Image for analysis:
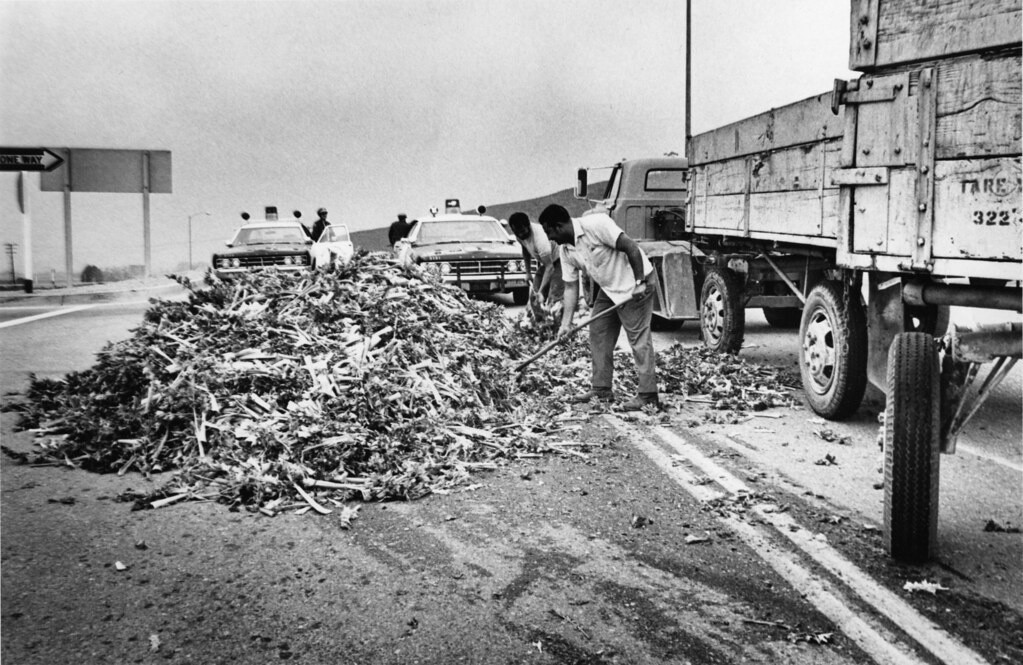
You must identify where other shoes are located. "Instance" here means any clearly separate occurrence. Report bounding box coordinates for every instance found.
[573,387,614,402]
[620,395,658,410]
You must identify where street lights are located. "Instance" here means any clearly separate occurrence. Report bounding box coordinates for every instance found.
[189,211,210,270]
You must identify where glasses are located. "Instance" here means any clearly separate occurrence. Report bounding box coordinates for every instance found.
[321,212,328,214]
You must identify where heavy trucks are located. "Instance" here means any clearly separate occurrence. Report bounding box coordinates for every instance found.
[575,89,951,423]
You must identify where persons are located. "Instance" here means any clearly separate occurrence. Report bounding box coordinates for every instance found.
[388,213,418,243]
[312,208,335,244]
[538,202,660,411]
[507,210,562,326]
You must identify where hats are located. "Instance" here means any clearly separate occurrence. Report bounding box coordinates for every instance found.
[317,208,327,214]
[398,213,407,218]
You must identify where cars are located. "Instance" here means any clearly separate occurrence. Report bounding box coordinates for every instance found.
[210,221,354,279]
[394,214,526,306]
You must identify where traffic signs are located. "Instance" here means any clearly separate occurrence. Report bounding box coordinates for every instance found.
[0,147,65,172]
